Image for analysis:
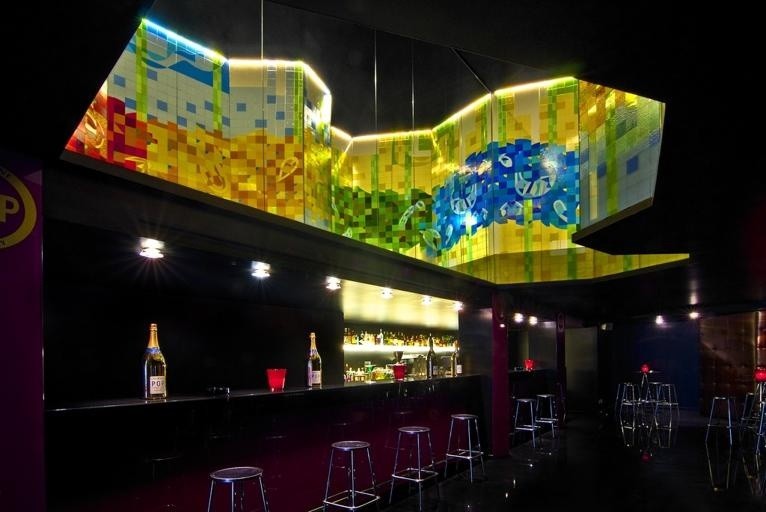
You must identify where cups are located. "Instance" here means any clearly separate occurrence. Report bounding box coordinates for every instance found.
[524,358,535,370]
[266,367,288,393]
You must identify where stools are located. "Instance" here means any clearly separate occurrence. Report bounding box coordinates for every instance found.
[706,391,766,436]
[323,411,486,511]
[615,381,680,416]
[513,393,561,448]
[207,466,269,512]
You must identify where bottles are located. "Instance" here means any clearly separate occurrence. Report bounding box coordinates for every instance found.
[305,331,323,387]
[140,323,168,400]
[342,325,461,383]
